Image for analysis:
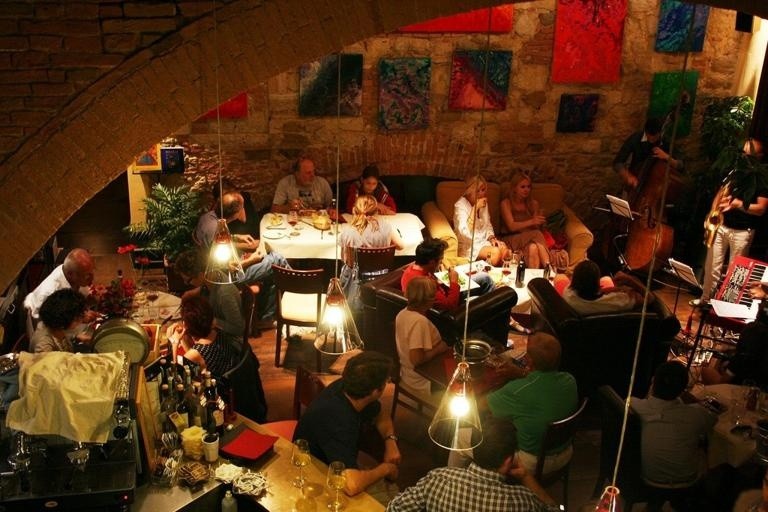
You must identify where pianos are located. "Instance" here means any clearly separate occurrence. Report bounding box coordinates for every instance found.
[708,255,768,327]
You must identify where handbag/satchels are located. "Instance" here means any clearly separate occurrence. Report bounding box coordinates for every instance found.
[554,230,569,251]
[545,208,568,230]
[542,230,555,251]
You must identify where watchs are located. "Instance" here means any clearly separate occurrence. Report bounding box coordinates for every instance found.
[233,234,236,241]
[169,336,179,344]
[383,434,398,441]
[71,332,78,345]
[519,368,528,377]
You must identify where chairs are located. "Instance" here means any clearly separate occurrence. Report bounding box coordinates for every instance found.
[533,397,589,512]
[173,249,263,289]
[596,384,704,511]
[235,283,256,346]
[128,244,165,280]
[257,364,327,443]
[270,263,327,367]
[345,244,396,283]
[389,359,437,429]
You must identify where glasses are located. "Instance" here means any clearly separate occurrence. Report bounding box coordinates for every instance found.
[183,277,194,287]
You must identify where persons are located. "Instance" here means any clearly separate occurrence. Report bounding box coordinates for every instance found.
[401,237,461,310]
[346,163,397,215]
[477,333,577,475]
[166,292,243,395]
[612,117,683,187]
[624,360,719,512]
[270,159,337,217]
[454,174,507,267]
[721,281,768,384]
[689,137,768,306]
[500,172,550,269]
[292,351,402,497]
[338,195,405,313]
[173,252,244,328]
[210,176,259,246]
[29,248,94,330]
[28,288,88,353]
[385,416,560,512]
[394,276,449,397]
[553,260,655,315]
[196,191,293,330]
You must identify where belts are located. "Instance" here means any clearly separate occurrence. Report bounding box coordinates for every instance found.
[519,440,580,457]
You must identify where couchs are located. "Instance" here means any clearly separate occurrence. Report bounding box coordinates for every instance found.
[525,272,679,398]
[358,260,517,352]
[422,181,593,271]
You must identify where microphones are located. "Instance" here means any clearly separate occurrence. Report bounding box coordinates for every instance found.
[722,168,739,183]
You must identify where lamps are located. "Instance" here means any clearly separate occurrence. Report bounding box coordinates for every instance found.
[313,47,361,354]
[592,1,699,510]
[426,3,493,451]
[203,6,245,284]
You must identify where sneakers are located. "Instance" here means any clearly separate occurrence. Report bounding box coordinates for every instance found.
[689,299,704,305]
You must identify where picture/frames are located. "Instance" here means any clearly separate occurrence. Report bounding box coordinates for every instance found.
[132,142,161,171]
[135,367,160,480]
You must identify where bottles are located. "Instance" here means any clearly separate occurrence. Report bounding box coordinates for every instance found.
[221,490,238,512]
[543,262,550,282]
[116,269,123,284]
[330,199,337,222]
[484,252,492,272]
[515,255,526,288]
[159,358,224,437]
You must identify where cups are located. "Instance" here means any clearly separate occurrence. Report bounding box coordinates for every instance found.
[287,210,298,236]
[289,438,311,491]
[145,284,160,310]
[325,461,347,511]
[501,261,511,284]
[202,433,220,465]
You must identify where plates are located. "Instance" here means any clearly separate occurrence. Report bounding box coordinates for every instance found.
[262,228,286,239]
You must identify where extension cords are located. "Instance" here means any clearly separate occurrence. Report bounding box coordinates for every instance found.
[695,352,713,364]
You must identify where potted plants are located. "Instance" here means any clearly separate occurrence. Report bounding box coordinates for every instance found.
[125,179,203,290]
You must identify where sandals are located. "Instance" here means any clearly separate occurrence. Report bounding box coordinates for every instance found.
[526,325,544,335]
[509,318,525,332]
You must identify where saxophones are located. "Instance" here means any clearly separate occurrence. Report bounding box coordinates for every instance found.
[705,182,732,248]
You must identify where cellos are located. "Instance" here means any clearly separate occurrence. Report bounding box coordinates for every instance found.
[625,91,690,270]
